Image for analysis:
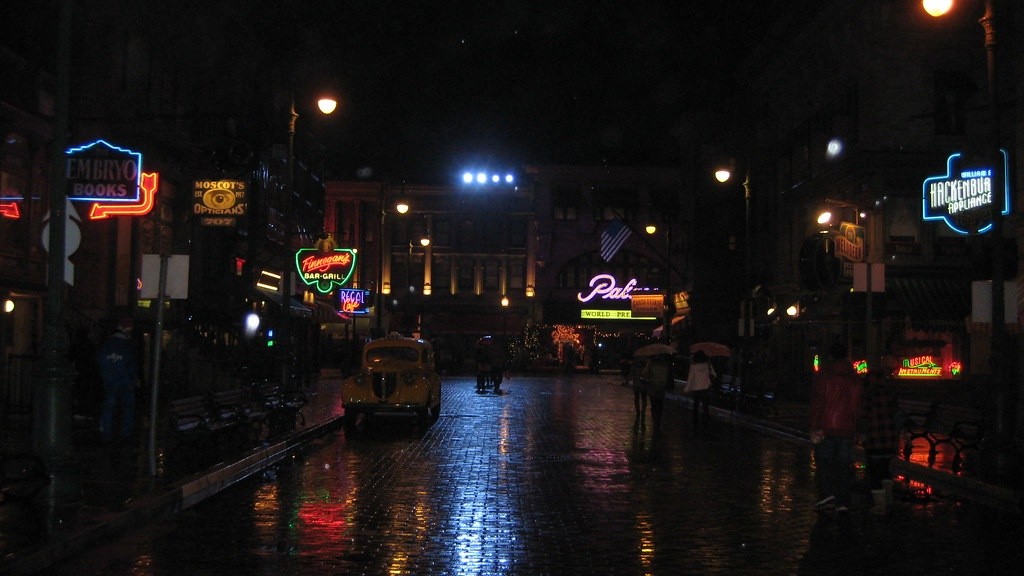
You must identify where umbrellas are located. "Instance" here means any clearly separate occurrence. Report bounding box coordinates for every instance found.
[689,342,732,363]
[633,343,678,361]
[478,336,496,343]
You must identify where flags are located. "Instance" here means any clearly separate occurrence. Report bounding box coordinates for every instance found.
[600,218,632,262]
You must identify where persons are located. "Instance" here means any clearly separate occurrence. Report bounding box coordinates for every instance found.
[96,316,144,454]
[683,350,717,435]
[473,341,506,395]
[627,354,651,423]
[640,351,678,429]
[808,352,860,513]
[855,366,901,516]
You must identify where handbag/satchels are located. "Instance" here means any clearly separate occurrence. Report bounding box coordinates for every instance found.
[666,373,675,393]
[708,361,720,393]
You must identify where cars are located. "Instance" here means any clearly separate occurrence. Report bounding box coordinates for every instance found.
[342,336,442,435]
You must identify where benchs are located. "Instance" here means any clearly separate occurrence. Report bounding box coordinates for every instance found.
[895,398,984,472]
[0,451,53,539]
[715,373,778,416]
[167,379,309,472]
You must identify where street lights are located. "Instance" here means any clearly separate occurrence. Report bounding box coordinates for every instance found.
[373,169,412,334]
[644,216,674,342]
[919,1,1012,480]
[714,150,754,378]
[282,76,339,410]
